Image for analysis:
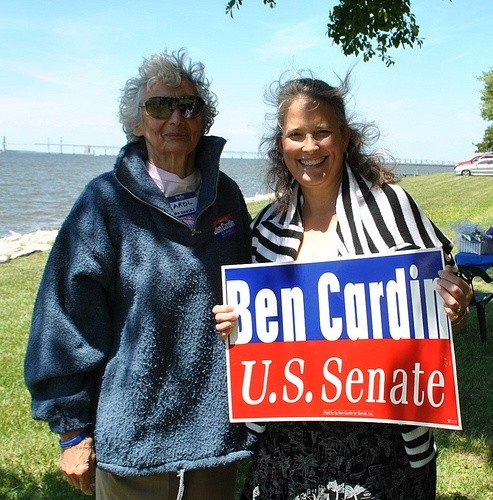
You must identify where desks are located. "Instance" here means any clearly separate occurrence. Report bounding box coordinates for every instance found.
[454,253,493,270]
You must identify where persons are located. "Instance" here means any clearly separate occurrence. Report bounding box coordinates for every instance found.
[211,62,474,500]
[22,47,260,500]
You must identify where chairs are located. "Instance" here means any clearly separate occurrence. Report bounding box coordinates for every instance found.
[455,266,493,344]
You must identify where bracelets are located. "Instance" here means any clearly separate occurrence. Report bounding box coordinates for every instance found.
[59,429,88,449]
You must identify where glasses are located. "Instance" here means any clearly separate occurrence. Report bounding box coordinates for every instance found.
[140,95,206,120]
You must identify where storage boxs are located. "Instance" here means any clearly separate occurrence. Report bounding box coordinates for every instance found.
[459,238,491,255]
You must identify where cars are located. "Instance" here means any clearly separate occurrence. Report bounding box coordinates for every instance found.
[455,155,493,165]
[455,159,493,177]
